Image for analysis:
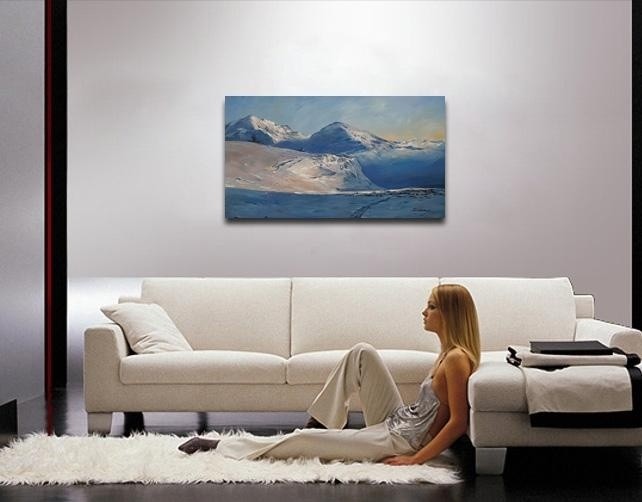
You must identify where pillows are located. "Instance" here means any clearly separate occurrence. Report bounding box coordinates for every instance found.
[99,298,194,358]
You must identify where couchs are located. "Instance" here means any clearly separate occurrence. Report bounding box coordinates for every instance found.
[80,273,641,474]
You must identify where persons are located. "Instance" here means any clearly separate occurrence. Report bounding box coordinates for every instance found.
[176,282,482,466]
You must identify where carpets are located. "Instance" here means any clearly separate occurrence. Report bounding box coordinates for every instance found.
[1,429,465,488]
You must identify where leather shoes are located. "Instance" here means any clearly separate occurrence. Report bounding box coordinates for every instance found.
[178,437,221,454]
